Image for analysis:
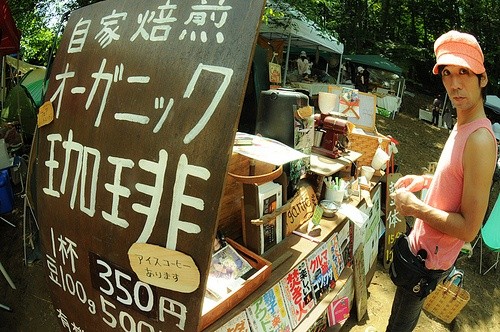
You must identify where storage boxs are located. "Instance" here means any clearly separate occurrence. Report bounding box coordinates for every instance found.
[0,168,14,213]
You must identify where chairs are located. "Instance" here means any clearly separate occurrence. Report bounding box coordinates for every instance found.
[472,190,500,275]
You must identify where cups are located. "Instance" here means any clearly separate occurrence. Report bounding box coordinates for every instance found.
[317,92,337,114]
[371,146,390,170]
[320,199,339,218]
[325,187,345,204]
[361,165,376,181]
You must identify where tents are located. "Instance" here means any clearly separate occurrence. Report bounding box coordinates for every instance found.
[484,95,500,114]
[258,0,344,86]
[341,55,404,120]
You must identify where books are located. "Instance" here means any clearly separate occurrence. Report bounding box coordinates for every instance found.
[213,233,350,332]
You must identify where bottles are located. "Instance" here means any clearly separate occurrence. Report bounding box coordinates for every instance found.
[258,87,310,149]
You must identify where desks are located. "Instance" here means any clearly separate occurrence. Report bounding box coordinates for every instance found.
[419,108,443,128]
[292,82,330,104]
[376,93,402,120]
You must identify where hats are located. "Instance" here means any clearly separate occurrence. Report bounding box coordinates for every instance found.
[300,51,306,57]
[433,30,486,75]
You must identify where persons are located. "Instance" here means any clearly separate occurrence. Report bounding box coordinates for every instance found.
[430,95,442,127]
[297,51,310,78]
[355,66,365,92]
[386,29,498,332]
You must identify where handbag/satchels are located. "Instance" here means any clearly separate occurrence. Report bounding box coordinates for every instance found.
[385,227,428,289]
[421,273,471,324]
